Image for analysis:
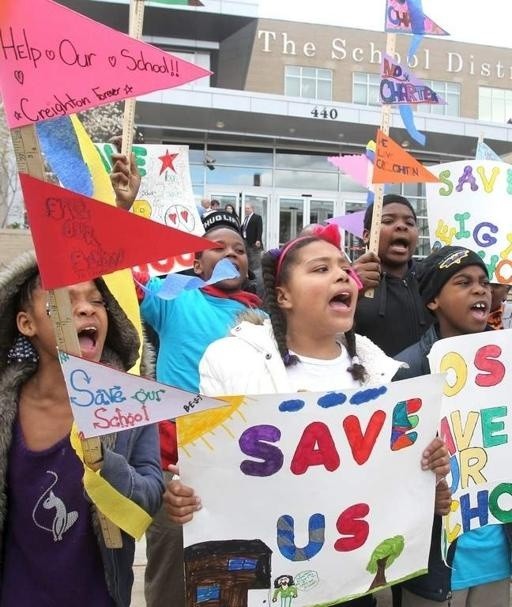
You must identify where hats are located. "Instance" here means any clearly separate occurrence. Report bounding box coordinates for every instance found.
[417,245,489,316]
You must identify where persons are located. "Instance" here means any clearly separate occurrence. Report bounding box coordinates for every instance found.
[391,246,512,607]
[109,153,270,607]
[300,194,512,358]
[0,249,166,607]
[163,236,450,607]
